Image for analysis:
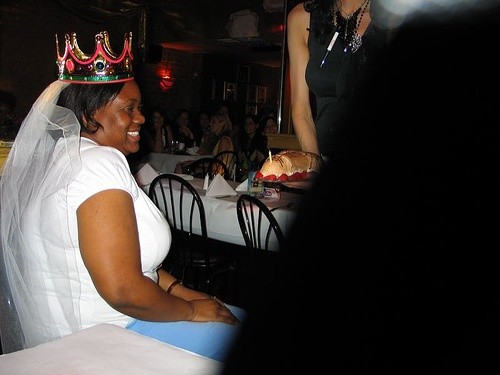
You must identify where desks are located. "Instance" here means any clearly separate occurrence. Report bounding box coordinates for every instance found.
[136,168,299,254]
[148,150,213,173]
[0,322,224,375]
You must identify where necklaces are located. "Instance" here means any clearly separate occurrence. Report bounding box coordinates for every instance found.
[332,0,370,50]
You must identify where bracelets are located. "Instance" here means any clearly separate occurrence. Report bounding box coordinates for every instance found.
[167,279,180,294]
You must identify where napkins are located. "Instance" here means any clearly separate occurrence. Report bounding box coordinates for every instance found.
[204,168,240,200]
[134,162,160,187]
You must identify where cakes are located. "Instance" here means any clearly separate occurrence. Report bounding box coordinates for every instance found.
[255,150,323,181]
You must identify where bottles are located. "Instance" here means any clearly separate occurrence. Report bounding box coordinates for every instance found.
[248,157,264,199]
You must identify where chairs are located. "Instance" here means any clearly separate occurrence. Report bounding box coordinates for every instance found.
[183,157,230,180]
[234,194,285,259]
[208,150,238,179]
[148,173,223,299]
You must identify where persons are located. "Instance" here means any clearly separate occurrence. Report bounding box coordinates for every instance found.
[0,90,26,139]
[0,78,246,363]
[145,0,500,375]
